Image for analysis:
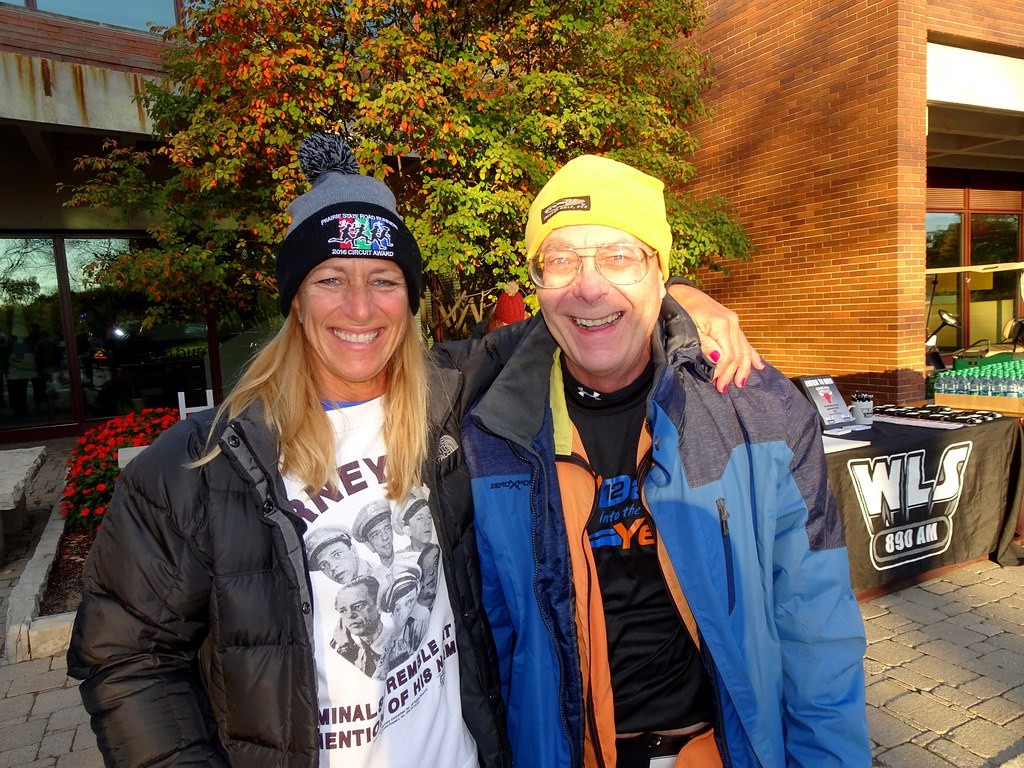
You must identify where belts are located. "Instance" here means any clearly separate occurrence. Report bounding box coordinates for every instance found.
[650,725,712,758]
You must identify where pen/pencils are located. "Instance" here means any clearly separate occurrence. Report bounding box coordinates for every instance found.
[851,390,873,402]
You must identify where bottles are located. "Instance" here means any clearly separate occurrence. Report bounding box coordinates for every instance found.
[934,359,1024,396]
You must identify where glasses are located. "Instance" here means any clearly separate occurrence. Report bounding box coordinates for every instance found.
[524,244,658,290]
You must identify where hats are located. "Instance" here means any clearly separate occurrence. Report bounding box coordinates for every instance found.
[526,155,672,284]
[275,133,423,316]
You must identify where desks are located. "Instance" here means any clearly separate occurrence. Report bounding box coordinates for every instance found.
[819,404,1024,604]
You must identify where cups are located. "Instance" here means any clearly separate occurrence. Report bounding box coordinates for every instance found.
[847,400,874,425]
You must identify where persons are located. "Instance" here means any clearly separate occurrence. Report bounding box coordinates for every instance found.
[66,172,765,768]
[460,154,873,768]
[471,293,532,339]
[0,320,103,421]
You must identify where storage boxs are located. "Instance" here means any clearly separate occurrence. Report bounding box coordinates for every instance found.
[934,393,1024,419]
